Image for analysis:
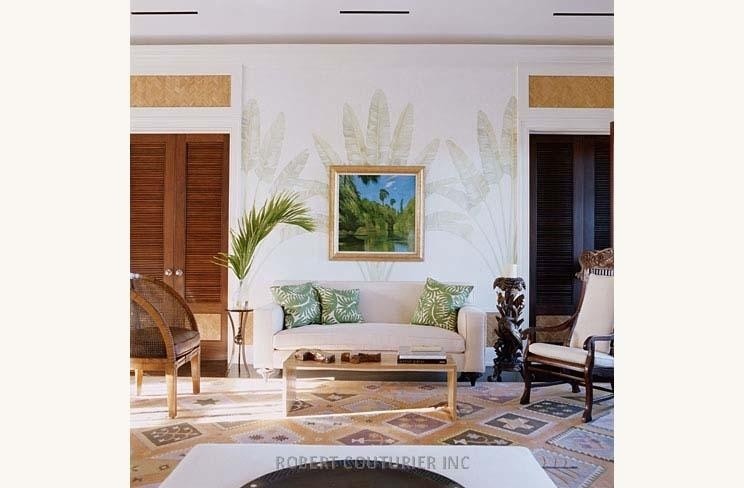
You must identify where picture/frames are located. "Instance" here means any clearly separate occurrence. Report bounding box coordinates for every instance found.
[329,165,425,262]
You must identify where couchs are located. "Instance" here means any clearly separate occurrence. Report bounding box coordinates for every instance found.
[251,280,487,386]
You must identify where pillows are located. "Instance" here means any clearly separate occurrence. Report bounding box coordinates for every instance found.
[310,282,365,324]
[270,283,322,329]
[409,277,474,333]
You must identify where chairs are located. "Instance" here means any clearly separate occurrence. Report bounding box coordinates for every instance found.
[516,247,614,424]
[130,272,202,420]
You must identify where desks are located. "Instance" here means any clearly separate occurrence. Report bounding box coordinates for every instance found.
[158,443,558,488]
[224,307,254,378]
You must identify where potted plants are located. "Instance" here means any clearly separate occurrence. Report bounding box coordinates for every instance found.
[206,187,320,309]
